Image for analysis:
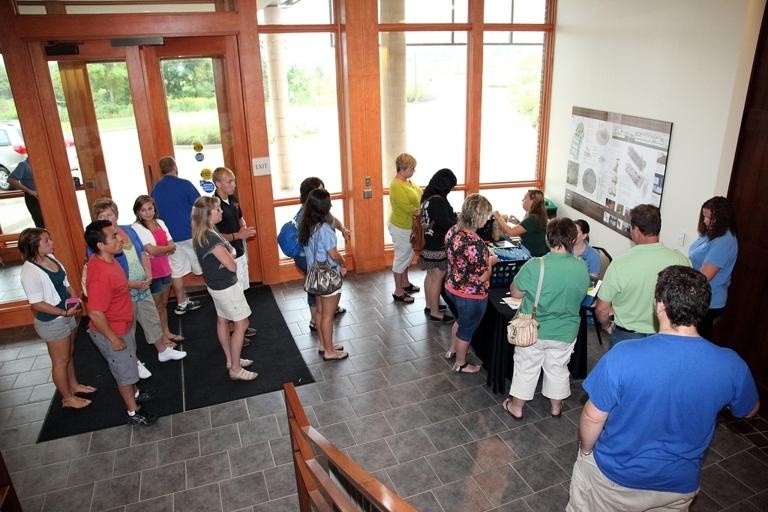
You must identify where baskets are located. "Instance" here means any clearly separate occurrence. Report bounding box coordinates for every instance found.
[489,245,533,287]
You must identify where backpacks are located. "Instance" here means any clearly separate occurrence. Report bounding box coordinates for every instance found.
[410,195,440,251]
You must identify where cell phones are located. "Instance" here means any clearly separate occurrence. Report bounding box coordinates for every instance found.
[67,302,81,310]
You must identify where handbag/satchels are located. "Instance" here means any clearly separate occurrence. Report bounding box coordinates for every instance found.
[505,258,545,348]
[303,236,343,296]
[277,208,305,258]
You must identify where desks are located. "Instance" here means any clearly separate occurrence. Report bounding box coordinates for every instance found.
[437,219,590,393]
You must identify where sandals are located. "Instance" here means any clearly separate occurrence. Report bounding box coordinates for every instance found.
[309,321,317,330]
[451,362,481,374]
[335,305,346,318]
[445,346,472,359]
[72,385,97,394]
[61,396,93,409]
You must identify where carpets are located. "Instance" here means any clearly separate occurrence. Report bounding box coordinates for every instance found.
[181,282,324,414]
[31,302,185,445]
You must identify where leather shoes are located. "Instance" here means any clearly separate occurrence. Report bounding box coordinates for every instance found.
[324,351,349,361]
[319,344,343,354]
[425,305,447,313]
[430,314,454,324]
[225,358,254,370]
[229,368,258,381]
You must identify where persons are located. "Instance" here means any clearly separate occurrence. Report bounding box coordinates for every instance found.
[595,205,693,350]
[131,195,185,350]
[85,220,158,425]
[388,153,423,303]
[492,190,550,257]
[86,198,187,379]
[420,169,459,324]
[212,167,257,347]
[298,188,348,361]
[502,217,589,420]
[151,157,204,316]
[688,197,739,345]
[7,157,46,227]
[444,194,497,374]
[18,228,97,408]
[191,197,258,382]
[573,220,601,325]
[294,177,352,332]
[566,265,760,512]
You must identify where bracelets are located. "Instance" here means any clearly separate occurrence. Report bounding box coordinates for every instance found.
[581,448,592,456]
[231,233,234,241]
[66,310,67,317]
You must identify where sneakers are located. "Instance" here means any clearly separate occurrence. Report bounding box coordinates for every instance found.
[174,298,202,314]
[135,387,158,404]
[136,359,153,380]
[123,407,157,426]
[245,325,257,336]
[157,348,187,362]
[243,337,252,350]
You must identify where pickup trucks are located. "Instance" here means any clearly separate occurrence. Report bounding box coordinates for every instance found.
[0,120,84,192]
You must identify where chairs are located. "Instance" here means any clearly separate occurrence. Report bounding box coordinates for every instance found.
[581,246,615,345]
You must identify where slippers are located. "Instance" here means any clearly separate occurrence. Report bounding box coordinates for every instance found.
[404,282,420,292]
[502,398,524,422]
[392,292,415,303]
[169,333,185,344]
[167,340,178,349]
[551,401,564,418]
